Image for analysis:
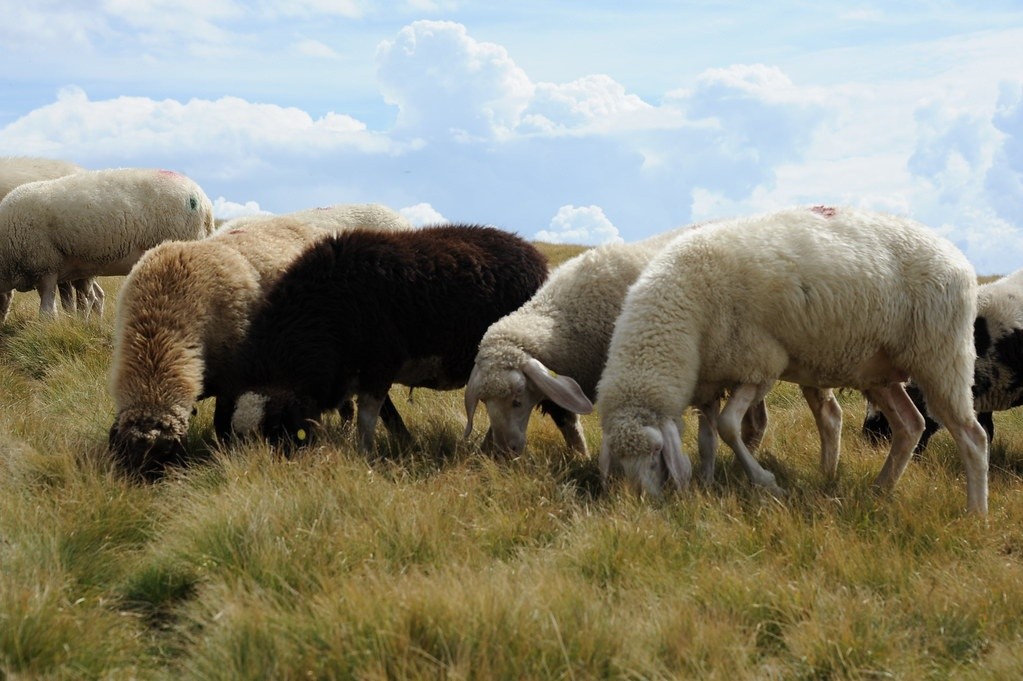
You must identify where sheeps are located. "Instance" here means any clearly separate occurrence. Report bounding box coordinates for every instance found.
[0,169,220,325]
[595,212,989,528]
[228,227,588,466]
[466,219,843,488]
[0,157,87,326]
[110,202,414,487]
[861,267,1023,466]
